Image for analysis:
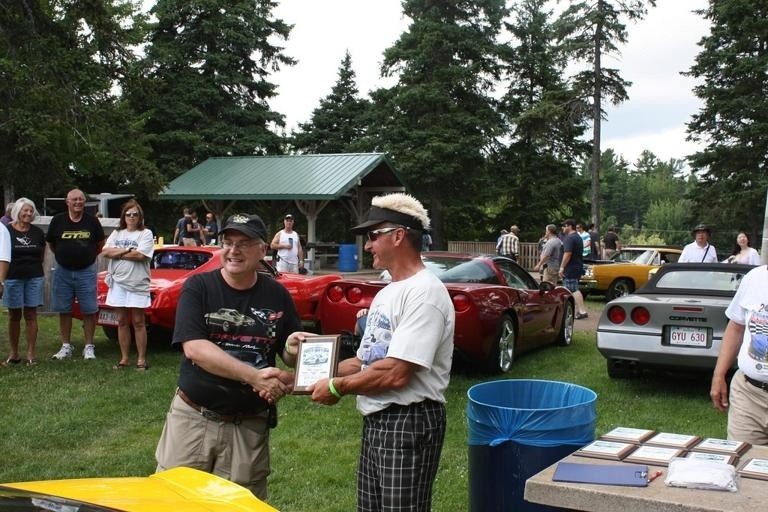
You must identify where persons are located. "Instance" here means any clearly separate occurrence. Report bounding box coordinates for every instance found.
[0,219,12,299]
[719,230,761,266]
[250,189,458,511]
[153,211,323,504]
[269,212,304,275]
[0,196,45,366]
[45,186,106,363]
[709,258,768,447]
[533,220,622,320]
[498,229,508,256]
[172,207,220,249]
[0,202,16,225]
[99,198,154,369]
[495,224,521,266]
[676,224,720,263]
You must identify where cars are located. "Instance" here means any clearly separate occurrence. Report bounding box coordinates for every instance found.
[0,465,281,511]
[556,242,684,301]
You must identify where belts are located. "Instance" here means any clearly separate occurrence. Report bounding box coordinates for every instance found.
[177,388,259,423]
[744,375,768,392]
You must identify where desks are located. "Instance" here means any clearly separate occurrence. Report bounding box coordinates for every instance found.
[522,426,768,512]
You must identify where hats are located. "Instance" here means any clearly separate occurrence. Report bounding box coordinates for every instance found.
[284,214,294,220]
[350,205,423,235]
[217,212,267,242]
[691,224,712,239]
[510,225,520,232]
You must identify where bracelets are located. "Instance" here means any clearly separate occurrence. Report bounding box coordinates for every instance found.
[298,258,304,264]
[283,337,290,353]
[126,245,134,253]
[0,280,5,286]
[328,377,343,399]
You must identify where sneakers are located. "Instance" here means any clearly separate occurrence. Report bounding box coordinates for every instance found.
[81,345,96,359]
[575,311,588,319]
[51,346,72,360]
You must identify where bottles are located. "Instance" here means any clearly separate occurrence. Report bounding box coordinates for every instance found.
[153,236,157,244]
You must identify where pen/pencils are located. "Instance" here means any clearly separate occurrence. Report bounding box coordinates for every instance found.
[648,471,662,482]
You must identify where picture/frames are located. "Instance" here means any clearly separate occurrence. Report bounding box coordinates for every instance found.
[572,427,768,481]
[291,334,342,396]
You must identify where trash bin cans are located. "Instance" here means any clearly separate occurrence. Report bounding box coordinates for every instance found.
[466,379,598,512]
[338,244,359,272]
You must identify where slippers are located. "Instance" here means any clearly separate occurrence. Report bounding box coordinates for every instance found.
[1,357,37,368]
[114,360,147,373]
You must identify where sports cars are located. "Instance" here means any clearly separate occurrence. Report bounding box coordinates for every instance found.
[592,262,762,381]
[66,240,346,350]
[307,249,581,377]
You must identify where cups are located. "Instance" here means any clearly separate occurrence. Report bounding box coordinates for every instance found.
[158,237,164,247]
[288,238,293,245]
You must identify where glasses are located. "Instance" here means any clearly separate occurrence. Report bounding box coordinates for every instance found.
[367,227,409,241]
[220,240,259,250]
[125,211,138,218]
